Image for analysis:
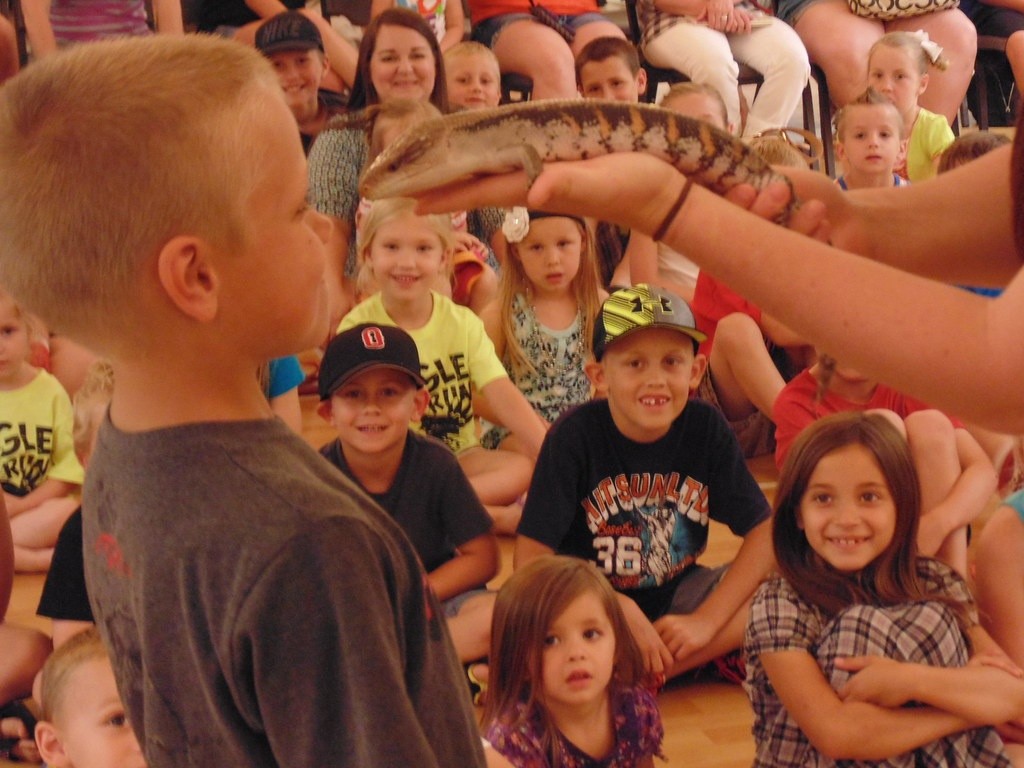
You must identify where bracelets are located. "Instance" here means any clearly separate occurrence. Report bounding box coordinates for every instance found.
[651,176,694,242]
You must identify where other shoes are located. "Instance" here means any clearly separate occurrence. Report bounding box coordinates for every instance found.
[465,664,482,704]
[675,649,747,686]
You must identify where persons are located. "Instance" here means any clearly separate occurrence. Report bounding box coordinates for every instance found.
[0,0,1024,768]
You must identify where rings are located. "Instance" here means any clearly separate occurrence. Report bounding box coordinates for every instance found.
[721,16,727,20]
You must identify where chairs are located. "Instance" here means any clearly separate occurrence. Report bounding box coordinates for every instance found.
[498,0,1024,185]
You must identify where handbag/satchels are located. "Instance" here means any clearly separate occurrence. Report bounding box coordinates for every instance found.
[685,11,772,27]
[848,0,959,21]
[532,3,576,41]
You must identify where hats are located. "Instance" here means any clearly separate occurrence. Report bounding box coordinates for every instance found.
[593,282,708,363]
[319,323,428,400]
[254,12,325,55]
